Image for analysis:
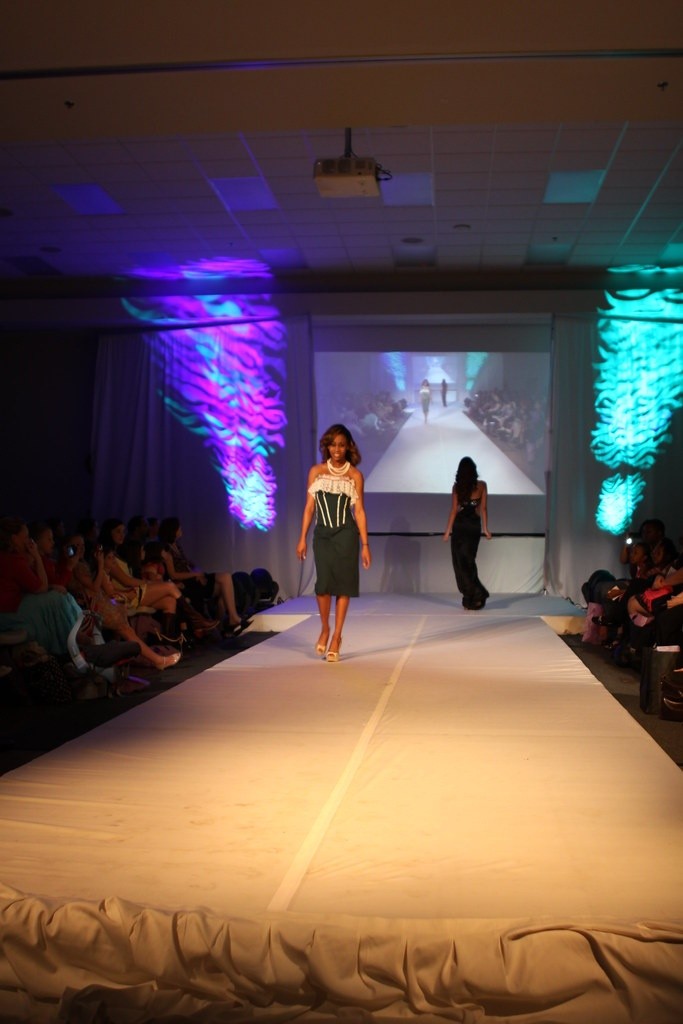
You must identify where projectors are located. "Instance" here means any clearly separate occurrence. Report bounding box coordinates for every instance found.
[313,157,381,198]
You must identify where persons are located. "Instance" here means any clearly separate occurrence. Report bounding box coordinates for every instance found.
[574,520,683,673]
[445,457,492,611]
[297,423,372,661]
[332,389,407,441]
[463,387,546,464]
[419,379,433,417]
[440,380,448,407]
[0,517,288,723]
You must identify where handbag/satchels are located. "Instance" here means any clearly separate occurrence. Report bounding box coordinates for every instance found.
[581,602,609,648]
[20,657,73,705]
[639,647,683,714]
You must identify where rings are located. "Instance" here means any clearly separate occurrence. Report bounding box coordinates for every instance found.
[608,591,611,594]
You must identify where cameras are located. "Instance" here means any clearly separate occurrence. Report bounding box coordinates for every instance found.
[627,532,641,544]
[66,545,77,557]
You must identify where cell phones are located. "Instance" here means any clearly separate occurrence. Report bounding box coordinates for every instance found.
[98,545,105,551]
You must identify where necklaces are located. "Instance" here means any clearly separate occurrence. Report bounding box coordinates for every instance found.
[327,458,350,476]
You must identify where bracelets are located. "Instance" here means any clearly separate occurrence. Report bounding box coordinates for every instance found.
[362,544,368,545]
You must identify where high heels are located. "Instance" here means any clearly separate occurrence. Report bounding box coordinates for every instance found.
[326,637,341,661]
[316,643,329,655]
[155,653,180,672]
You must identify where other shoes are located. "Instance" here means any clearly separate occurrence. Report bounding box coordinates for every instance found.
[187,614,220,634]
[230,619,254,636]
[608,642,624,651]
[1,666,11,677]
[592,615,614,627]
